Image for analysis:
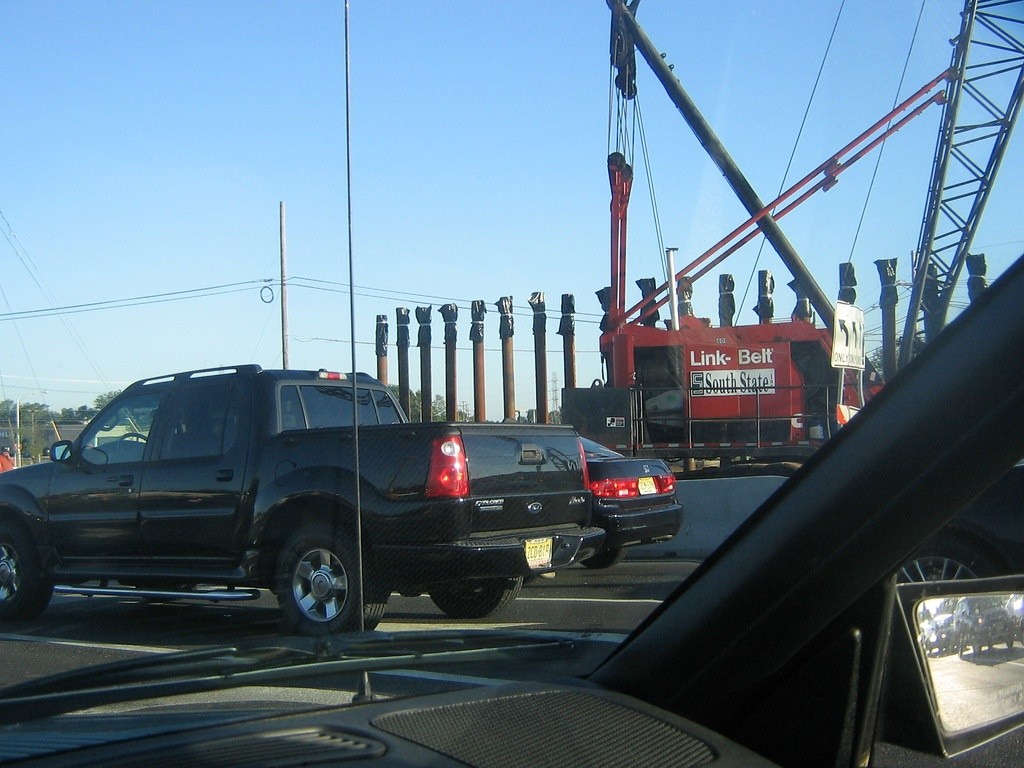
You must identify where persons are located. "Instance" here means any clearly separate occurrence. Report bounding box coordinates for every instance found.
[0,447,14,473]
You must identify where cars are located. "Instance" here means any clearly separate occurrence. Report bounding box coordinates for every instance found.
[575,435,683,570]
[917,593,1024,655]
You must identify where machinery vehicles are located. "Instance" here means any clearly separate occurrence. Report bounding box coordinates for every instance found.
[604,0,884,461]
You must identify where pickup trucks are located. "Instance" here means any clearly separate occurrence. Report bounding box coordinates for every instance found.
[1,364,607,637]
